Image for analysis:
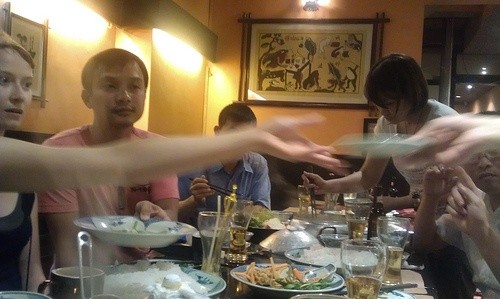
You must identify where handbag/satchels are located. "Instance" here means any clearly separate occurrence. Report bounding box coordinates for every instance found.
[427,246,475,299]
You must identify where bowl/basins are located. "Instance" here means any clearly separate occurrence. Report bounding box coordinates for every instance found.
[72,216,197,248]
[232,212,310,244]
[318,233,349,248]
[269,211,295,222]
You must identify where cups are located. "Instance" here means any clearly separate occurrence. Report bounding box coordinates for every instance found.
[197,199,254,277]
[340,239,387,299]
[298,181,412,291]
[0,290,53,299]
[37,266,122,299]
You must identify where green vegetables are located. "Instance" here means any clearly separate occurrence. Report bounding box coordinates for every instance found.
[233,212,263,228]
[277,268,335,290]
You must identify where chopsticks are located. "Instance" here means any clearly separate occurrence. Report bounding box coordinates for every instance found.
[189,177,244,200]
[310,177,317,217]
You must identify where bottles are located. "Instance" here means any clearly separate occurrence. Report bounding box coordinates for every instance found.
[367,186,386,240]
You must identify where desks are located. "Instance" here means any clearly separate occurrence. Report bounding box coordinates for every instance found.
[220,208,435,299]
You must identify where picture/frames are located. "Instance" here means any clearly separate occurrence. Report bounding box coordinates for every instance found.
[363,118,378,137]
[9,12,49,108]
[236,10,389,108]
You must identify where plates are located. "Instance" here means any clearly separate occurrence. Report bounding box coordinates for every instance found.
[284,246,382,276]
[331,138,425,157]
[230,264,417,299]
[179,266,227,296]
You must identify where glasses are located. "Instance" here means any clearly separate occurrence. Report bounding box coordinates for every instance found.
[466,151,500,165]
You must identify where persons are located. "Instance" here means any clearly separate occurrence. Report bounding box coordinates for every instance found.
[412,111,500,299]
[0,33,49,299]
[406,114,500,168]
[35,48,180,269]
[446,166,500,282]
[301,54,462,216]
[0,117,349,193]
[176,103,272,239]
